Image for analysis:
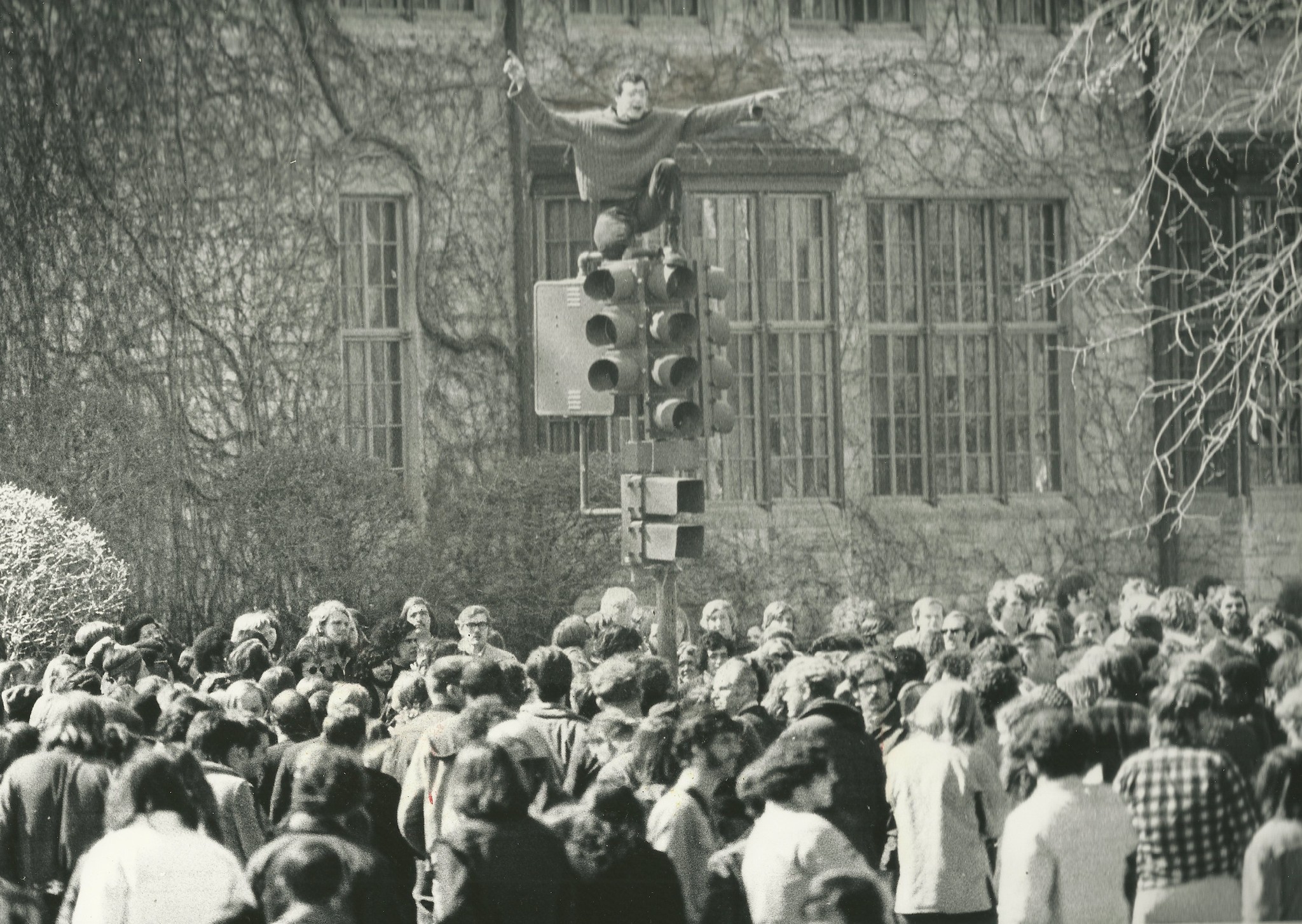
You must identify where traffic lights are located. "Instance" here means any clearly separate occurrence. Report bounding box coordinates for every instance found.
[583,269,639,392]
[646,262,700,435]
[642,476,706,561]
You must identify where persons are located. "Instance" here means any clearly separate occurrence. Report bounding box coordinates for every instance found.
[890,676,1000,922]
[1239,743,1302,920]
[743,572,1302,870]
[503,47,790,266]
[643,709,740,923]
[54,745,261,924]
[424,744,586,922]
[998,712,1138,920]
[573,778,687,924]
[1,690,111,922]
[1114,679,1259,922]
[179,712,263,873]
[2,582,736,856]
[238,743,414,924]
[268,835,353,924]
[738,734,898,924]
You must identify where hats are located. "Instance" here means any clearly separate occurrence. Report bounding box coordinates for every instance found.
[588,654,640,702]
[737,735,831,803]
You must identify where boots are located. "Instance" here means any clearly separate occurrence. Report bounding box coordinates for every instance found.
[659,213,689,268]
